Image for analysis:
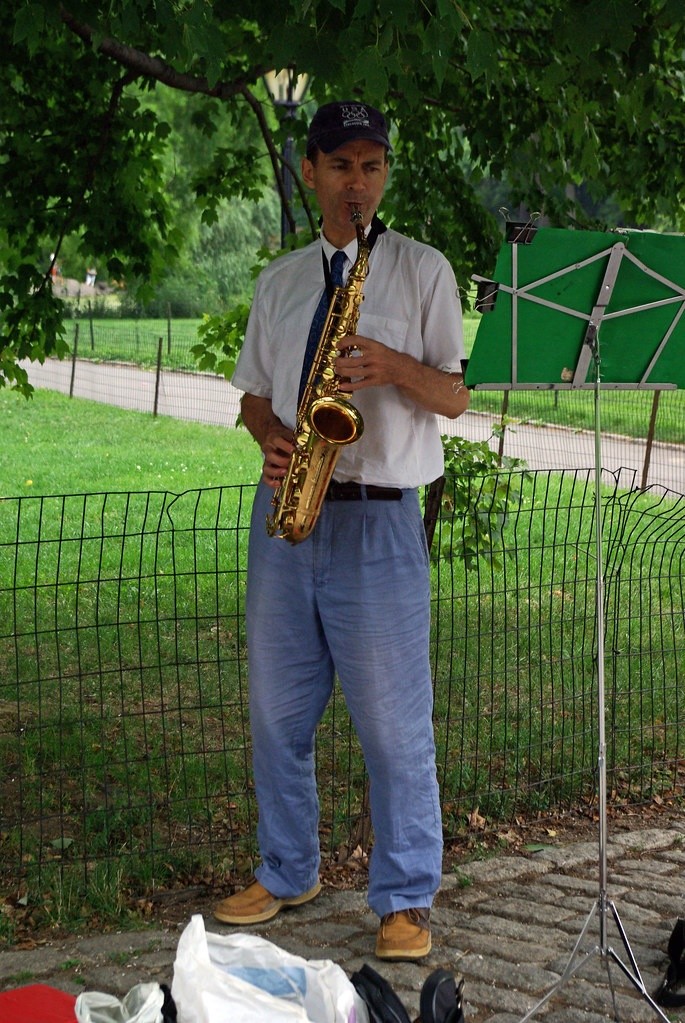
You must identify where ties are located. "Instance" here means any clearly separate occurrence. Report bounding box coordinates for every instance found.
[298,250,348,420]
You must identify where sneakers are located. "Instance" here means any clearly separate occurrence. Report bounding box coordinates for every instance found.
[214,870,321,925]
[374,907,432,960]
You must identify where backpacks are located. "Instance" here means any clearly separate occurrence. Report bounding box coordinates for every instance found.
[351,964,411,1023]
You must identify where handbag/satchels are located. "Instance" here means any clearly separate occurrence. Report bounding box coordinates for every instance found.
[419,967,466,1023]
[74,982,164,1023]
[656,917,685,1007]
[170,915,370,1023]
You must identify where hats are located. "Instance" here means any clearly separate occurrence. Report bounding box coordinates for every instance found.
[305,101,393,159]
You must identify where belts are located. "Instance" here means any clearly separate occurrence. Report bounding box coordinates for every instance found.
[325,480,403,500]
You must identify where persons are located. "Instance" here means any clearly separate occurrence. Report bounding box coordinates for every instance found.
[216,99,471,963]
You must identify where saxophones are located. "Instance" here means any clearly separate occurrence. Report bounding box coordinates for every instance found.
[266,201,387,544]
